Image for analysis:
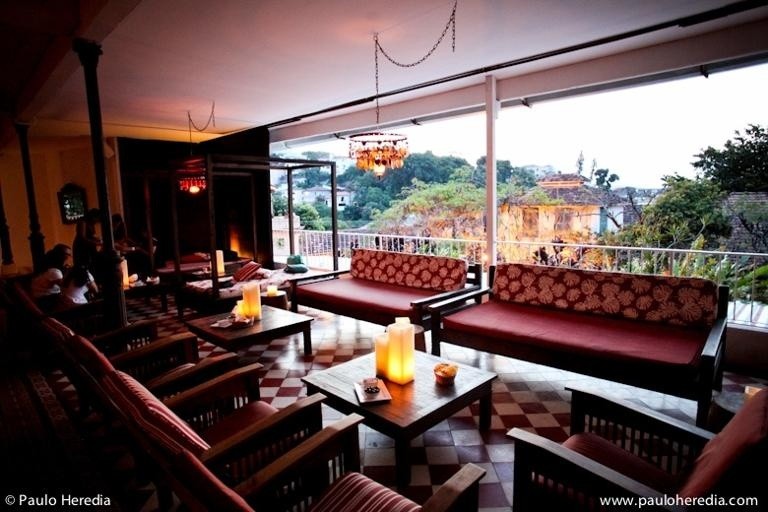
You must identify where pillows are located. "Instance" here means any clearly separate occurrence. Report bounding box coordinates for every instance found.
[284,252,309,274]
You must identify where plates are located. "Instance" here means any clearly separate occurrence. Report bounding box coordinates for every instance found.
[211,321,232,328]
[353,379,393,404]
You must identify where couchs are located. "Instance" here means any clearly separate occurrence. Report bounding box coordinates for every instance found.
[285,245,733,427]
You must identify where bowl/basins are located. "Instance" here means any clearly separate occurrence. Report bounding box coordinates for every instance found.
[434,363,455,386]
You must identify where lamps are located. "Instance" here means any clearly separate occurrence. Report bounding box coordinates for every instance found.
[174,102,217,198]
[346,2,460,180]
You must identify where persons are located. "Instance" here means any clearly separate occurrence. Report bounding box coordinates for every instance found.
[73,208,160,270]
[30,243,99,312]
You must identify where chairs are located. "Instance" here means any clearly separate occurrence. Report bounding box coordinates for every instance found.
[502,374,768,511]
[13,278,497,510]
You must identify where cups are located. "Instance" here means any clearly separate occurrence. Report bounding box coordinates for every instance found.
[363,377,377,388]
